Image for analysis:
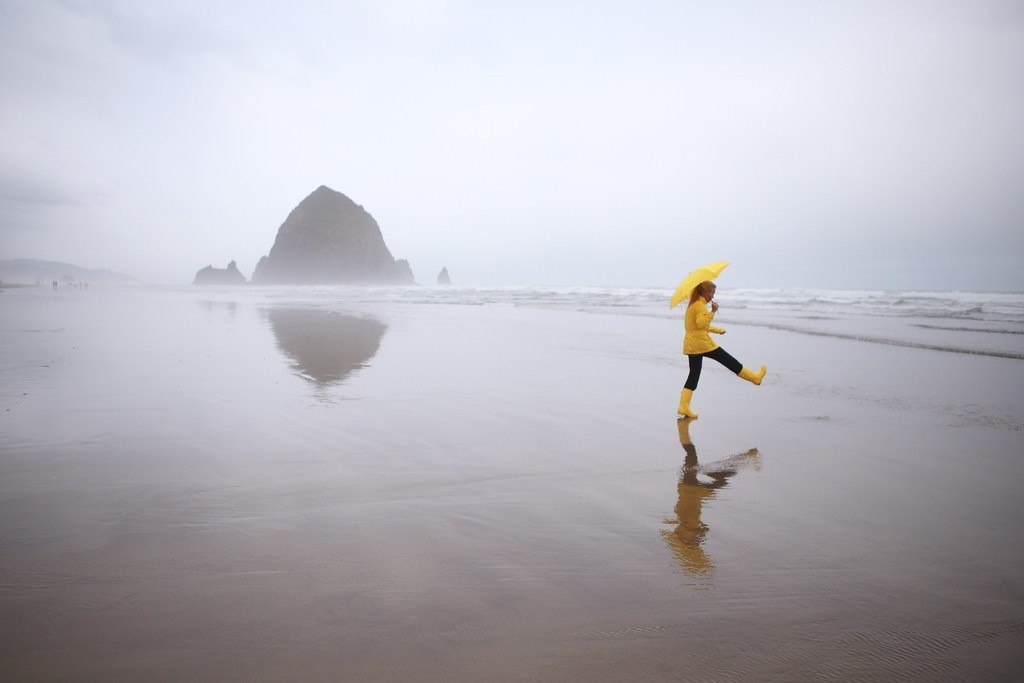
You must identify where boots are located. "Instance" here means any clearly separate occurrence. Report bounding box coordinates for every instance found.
[739,365,767,386]
[677,387,698,418]
[677,416,692,446]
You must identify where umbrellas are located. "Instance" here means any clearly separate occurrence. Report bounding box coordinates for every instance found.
[670,260,730,308]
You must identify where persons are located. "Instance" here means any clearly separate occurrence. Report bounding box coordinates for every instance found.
[677,281,766,418]
